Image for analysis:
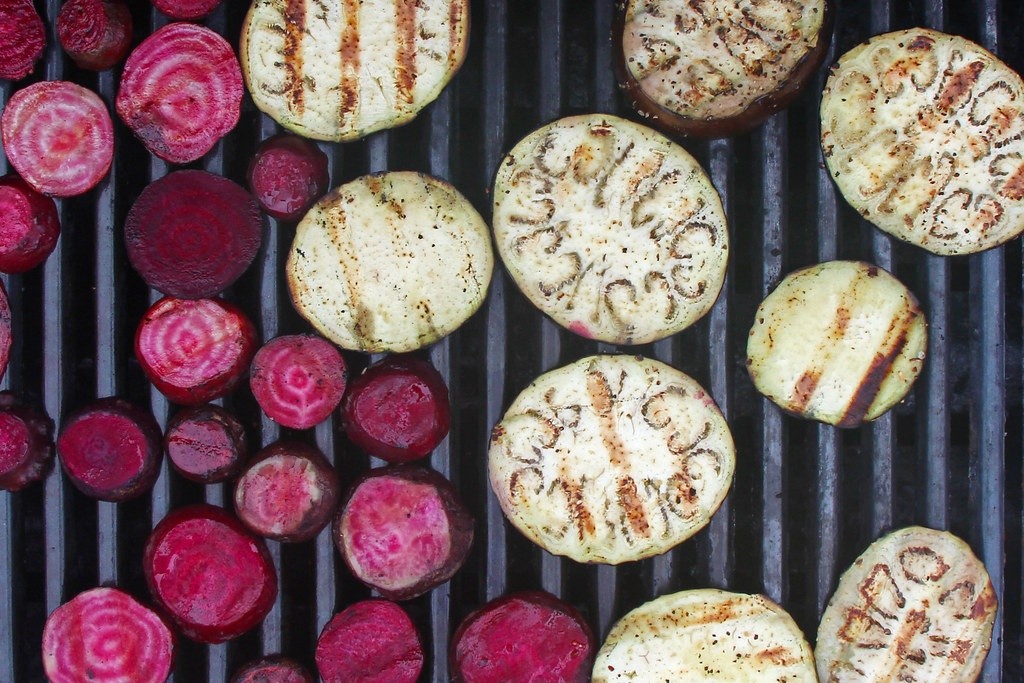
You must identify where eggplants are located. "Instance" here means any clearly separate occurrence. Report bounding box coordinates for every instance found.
[239,0,1024,683]
